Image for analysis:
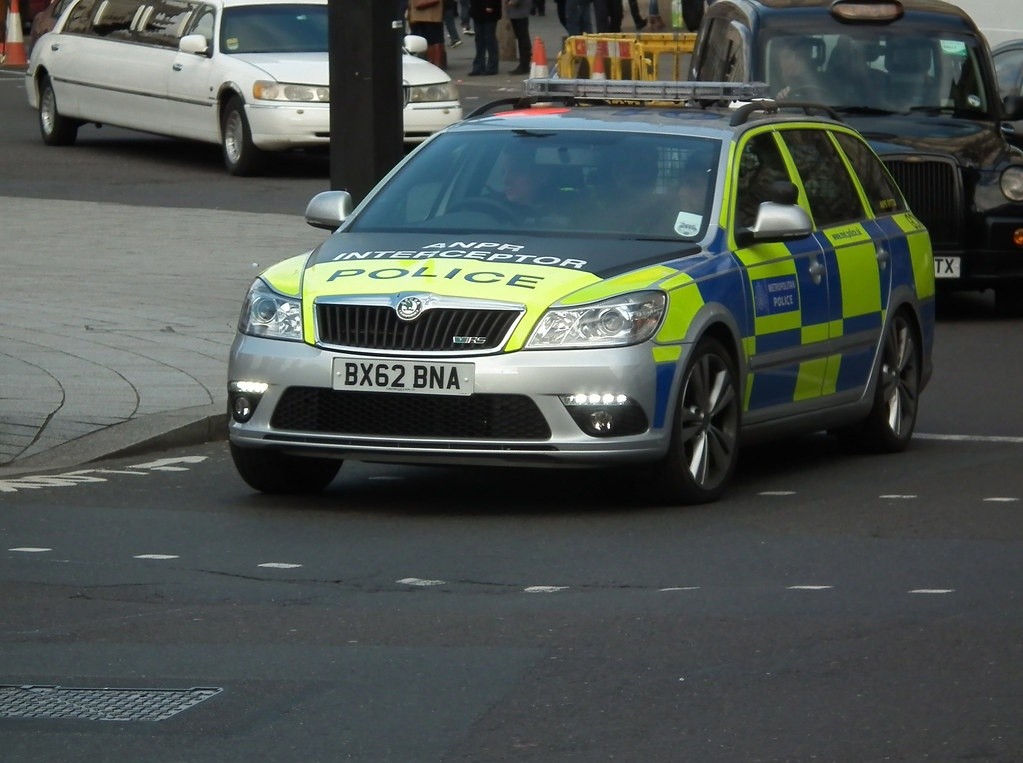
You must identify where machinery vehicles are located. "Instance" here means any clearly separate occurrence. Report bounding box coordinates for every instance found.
[495,32,697,196]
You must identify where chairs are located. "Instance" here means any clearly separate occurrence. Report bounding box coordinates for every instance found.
[883,41,940,109]
[548,166,584,205]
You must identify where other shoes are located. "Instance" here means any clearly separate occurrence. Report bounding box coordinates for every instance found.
[635,19,647,30]
[462,27,475,34]
[481,70,497,75]
[451,38,462,49]
[511,67,530,75]
[468,69,481,77]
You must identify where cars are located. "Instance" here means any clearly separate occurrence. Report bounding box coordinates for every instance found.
[682,1,1022,315]
[25,1,464,177]
[227,79,935,505]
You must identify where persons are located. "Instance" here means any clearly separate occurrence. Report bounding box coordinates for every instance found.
[464,143,572,227]
[554,0,624,35]
[773,39,828,100]
[680,0,706,31]
[638,150,717,233]
[467,0,502,75]
[409,0,443,67]
[504,0,532,74]
[627,0,667,30]
[460,0,473,30]
[443,0,461,48]
[531,0,545,16]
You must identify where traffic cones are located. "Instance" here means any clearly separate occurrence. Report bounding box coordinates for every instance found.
[520,37,552,106]
[588,54,607,77]
[0,0,28,67]
